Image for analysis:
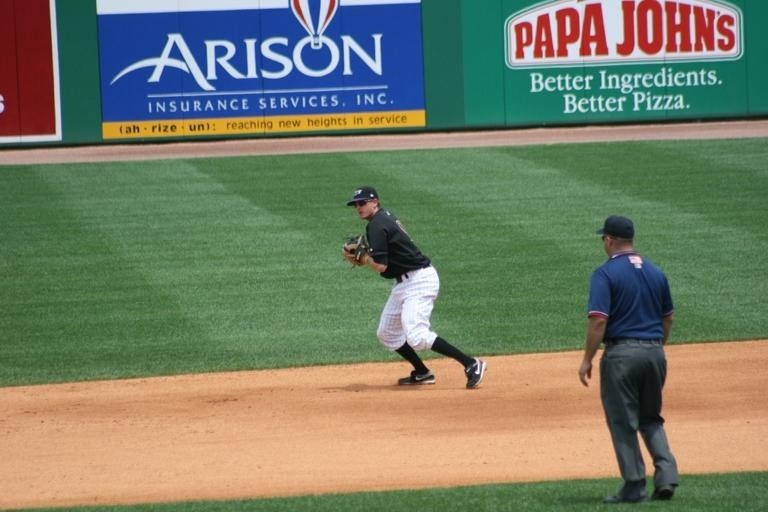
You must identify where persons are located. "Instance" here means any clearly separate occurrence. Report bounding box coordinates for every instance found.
[578,214,679,501]
[339,186,486,388]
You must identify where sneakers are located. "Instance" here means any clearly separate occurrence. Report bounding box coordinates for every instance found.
[465,358,487,388]
[399,369,435,385]
[652,484,674,499]
[604,482,647,502]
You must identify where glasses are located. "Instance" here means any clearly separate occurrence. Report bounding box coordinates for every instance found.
[354,200,372,207]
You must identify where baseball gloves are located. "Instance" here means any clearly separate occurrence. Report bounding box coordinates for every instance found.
[342,234,367,265]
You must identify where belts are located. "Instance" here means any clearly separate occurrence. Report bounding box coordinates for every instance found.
[606,339,664,344]
[396,273,408,283]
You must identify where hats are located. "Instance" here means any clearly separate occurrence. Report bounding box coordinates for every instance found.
[597,216,634,239]
[347,186,378,206]
